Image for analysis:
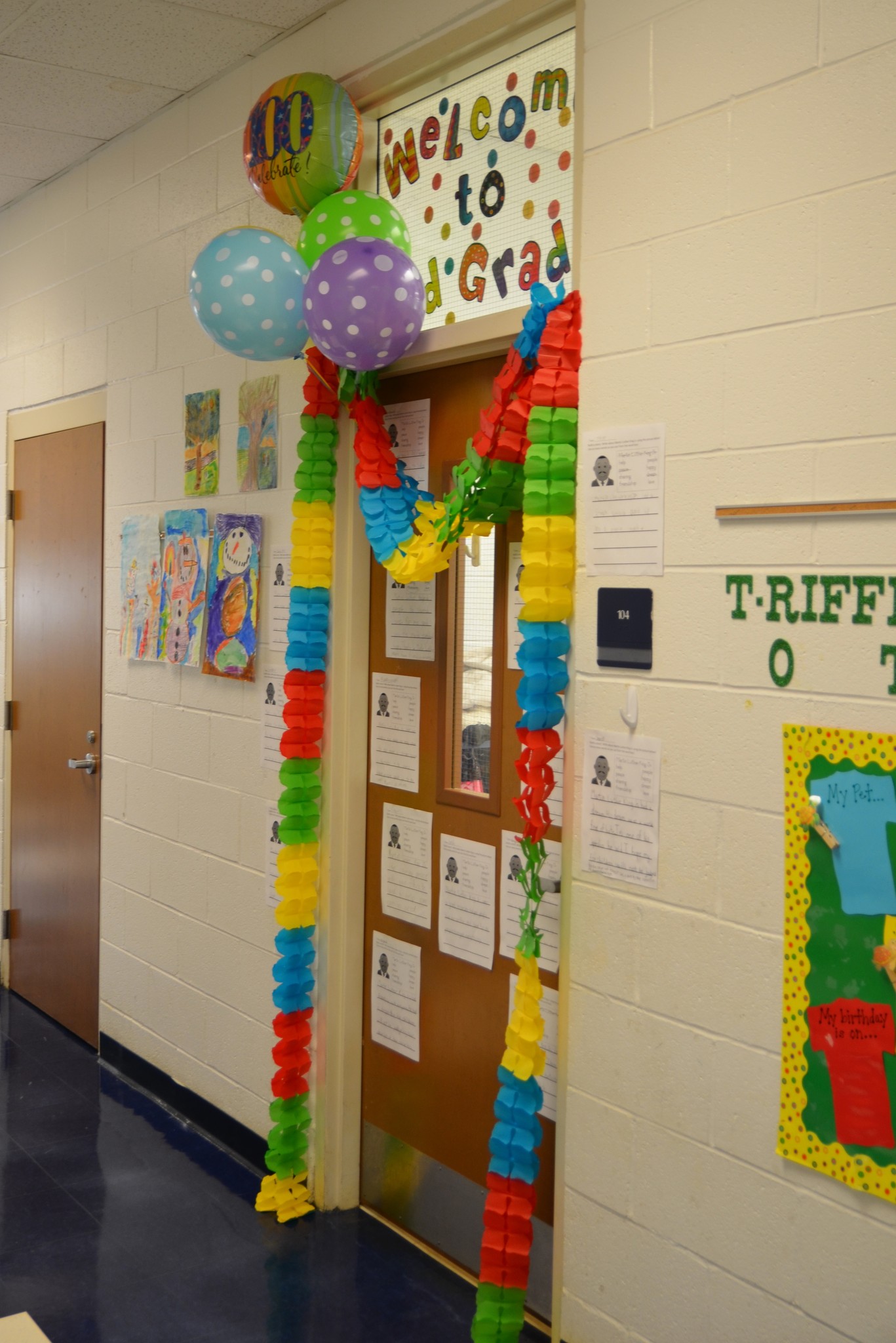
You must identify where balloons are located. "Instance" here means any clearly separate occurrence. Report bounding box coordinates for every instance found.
[243,73,365,224]
[190,226,310,362]
[304,237,425,372]
[296,189,411,270]
